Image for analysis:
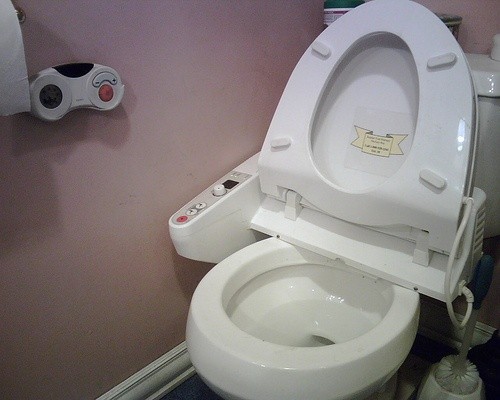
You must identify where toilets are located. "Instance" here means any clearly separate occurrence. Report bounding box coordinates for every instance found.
[168,0,500,400]
